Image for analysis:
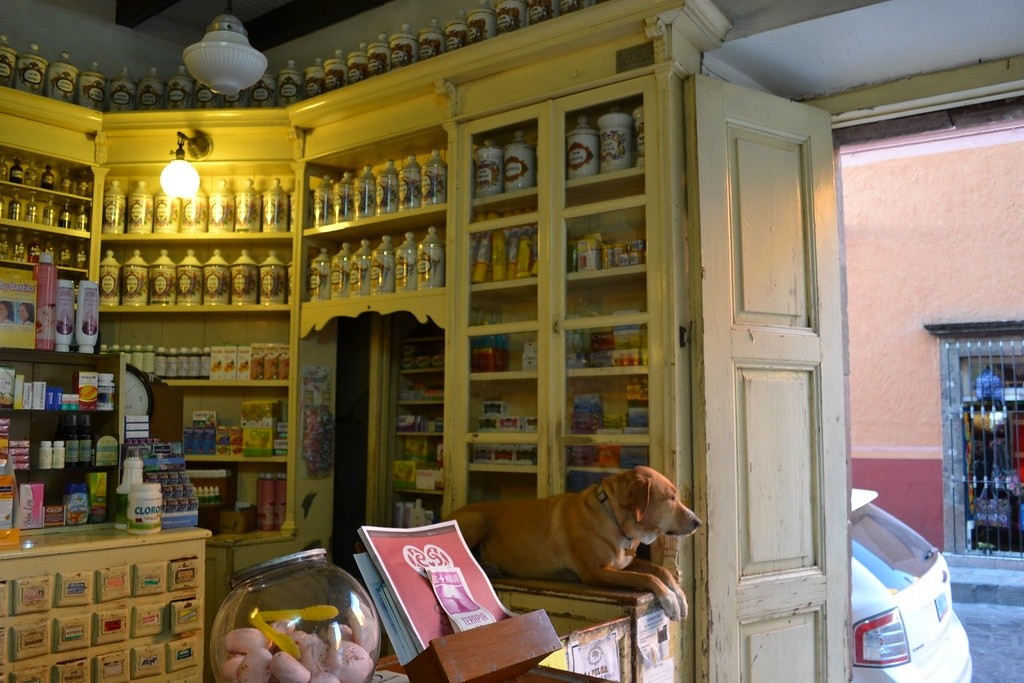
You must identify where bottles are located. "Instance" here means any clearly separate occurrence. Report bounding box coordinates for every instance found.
[37,412,166,539]
[0,0,650,380]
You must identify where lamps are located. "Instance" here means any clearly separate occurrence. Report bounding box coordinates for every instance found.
[159,132,213,198]
[183,0,268,96]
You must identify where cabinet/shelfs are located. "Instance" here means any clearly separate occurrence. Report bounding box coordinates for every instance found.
[0,0,734,683]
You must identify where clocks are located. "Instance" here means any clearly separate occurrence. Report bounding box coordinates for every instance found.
[125,362,153,415]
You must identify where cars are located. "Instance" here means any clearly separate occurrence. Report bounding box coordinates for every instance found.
[849,487,973,683]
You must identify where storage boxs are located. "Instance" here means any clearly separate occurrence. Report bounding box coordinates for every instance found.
[393,316,650,489]
[210,346,251,380]
[0,366,15,408]
[17,472,107,529]
[72,371,98,410]
[183,399,279,457]
[220,506,257,533]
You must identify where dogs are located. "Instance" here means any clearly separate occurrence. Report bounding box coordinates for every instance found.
[444,465,703,622]
